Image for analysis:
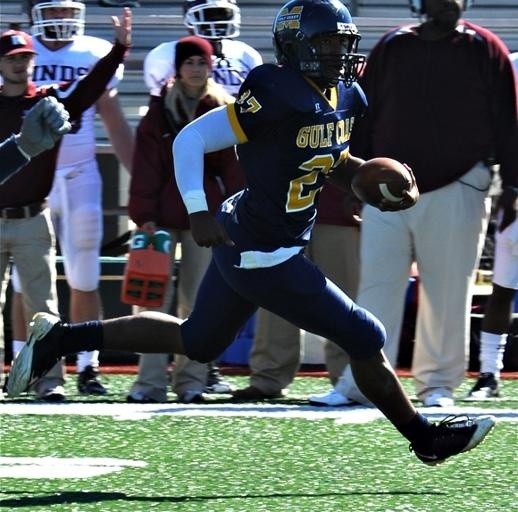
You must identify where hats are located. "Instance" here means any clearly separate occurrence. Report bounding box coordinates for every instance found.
[175,35,213,69]
[0,29,35,57]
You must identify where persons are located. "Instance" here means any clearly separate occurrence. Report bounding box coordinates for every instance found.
[0,1,261,403]
[5,0,496,466]
[233,0,518,406]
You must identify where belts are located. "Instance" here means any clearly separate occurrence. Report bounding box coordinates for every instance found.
[0,199,46,219]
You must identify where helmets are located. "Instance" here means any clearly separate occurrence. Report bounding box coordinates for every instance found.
[272,0,367,89]
[410,0,468,14]
[28,0,86,41]
[182,0,241,40]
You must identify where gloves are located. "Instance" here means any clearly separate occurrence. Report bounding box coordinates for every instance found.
[15,95,73,158]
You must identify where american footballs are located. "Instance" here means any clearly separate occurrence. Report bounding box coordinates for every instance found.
[351,157,412,203]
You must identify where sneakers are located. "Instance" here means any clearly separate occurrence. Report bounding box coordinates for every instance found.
[7,311,61,398]
[408,414,496,466]
